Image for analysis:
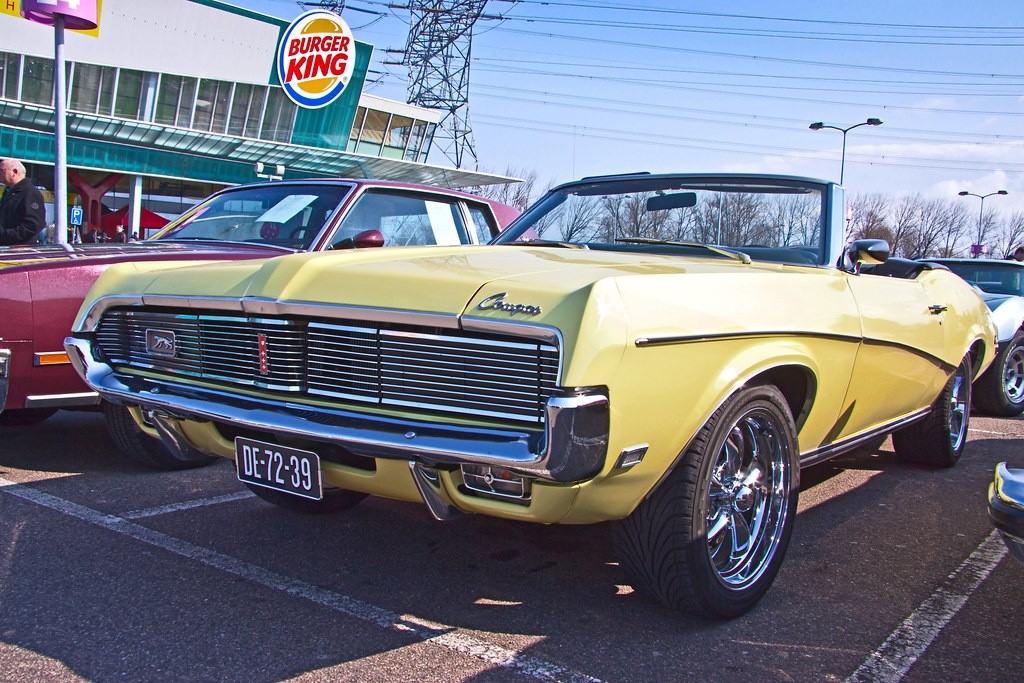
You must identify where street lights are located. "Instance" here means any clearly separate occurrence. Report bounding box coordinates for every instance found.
[601,195,632,242]
[809,118,884,186]
[959,191,1008,258]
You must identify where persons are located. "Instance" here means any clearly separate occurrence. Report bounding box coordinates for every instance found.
[1001,246,1024,290]
[38,221,55,246]
[86,227,107,243]
[129,232,139,241]
[67,224,74,242]
[0,159,47,246]
[111,225,126,243]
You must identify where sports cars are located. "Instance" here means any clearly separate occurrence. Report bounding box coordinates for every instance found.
[62,171,1000,625]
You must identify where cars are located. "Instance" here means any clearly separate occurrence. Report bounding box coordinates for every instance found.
[0,178,541,471]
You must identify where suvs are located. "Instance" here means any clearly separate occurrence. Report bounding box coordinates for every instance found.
[914,258,1024,417]
